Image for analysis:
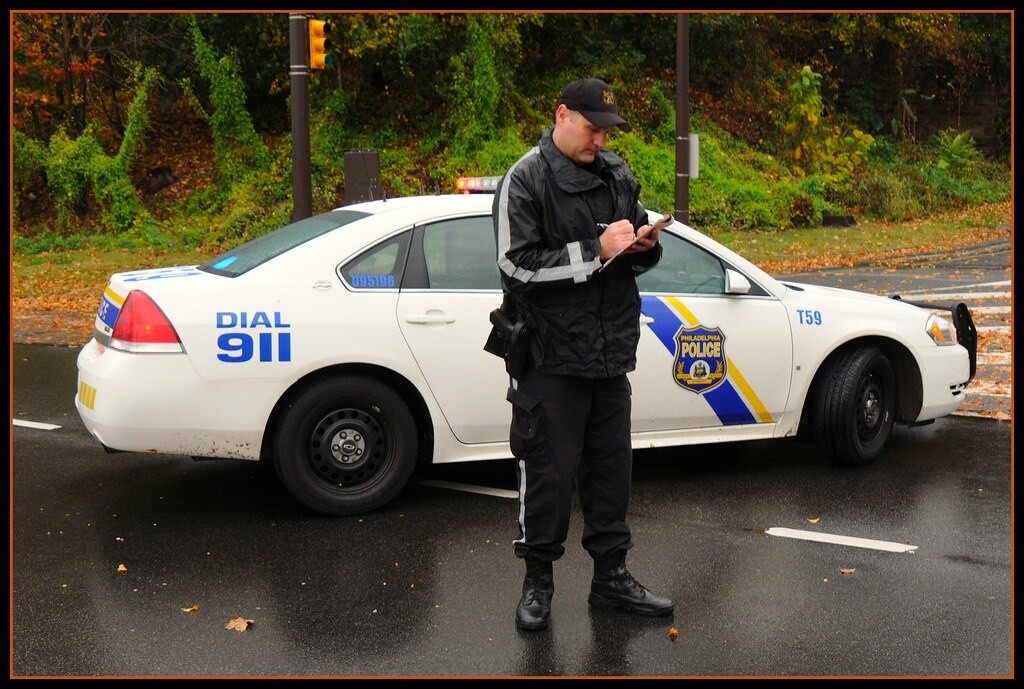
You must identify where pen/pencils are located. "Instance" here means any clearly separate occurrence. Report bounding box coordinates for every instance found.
[596,223,637,241]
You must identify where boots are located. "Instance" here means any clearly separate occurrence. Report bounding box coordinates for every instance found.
[515,557,555,631]
[588,555,674,616]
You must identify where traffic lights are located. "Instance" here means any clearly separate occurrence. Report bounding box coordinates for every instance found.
[308,18,333,71]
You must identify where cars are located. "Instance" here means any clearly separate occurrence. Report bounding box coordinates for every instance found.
[74,176,980,520]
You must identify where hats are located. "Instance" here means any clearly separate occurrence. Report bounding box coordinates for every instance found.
[558,78,627,128]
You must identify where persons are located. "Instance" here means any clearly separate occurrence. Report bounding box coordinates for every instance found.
[493,78,674,631]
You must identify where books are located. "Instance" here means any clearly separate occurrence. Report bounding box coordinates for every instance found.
[599,212,673,274]
